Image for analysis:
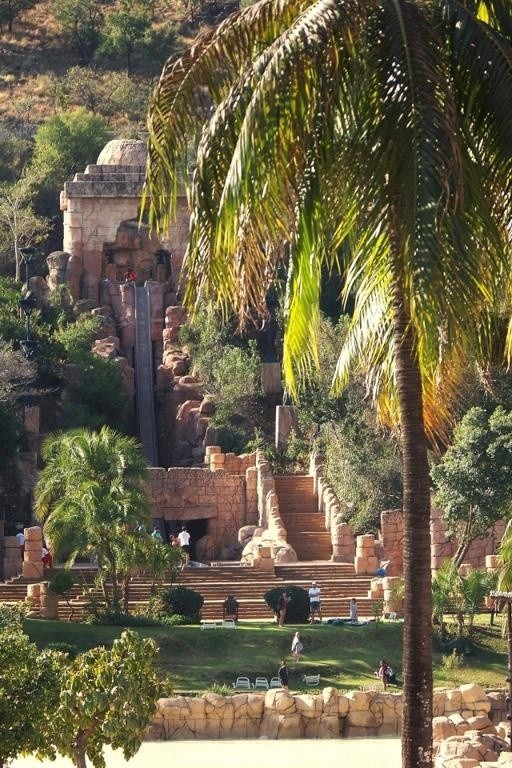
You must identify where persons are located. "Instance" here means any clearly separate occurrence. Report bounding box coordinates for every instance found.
[152,526,164,543]
[384,662,399,685]
[379,659,388,690]
[277,660,290,689]
[40,547,52,568]
[14,529,24,562]
[276,590,292,627]
[349,596,359,622]
[307,580,324,624]
[291,630,302,662]
[122,266,137,286]
[169,533,179,547]
[176,525,192,568]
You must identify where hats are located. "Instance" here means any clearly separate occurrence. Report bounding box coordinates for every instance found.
[312,582,317,585]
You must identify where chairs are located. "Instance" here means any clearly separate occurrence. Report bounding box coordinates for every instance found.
[220,619,236,630]
[389,612,396,623]
[255,676,269,689]
[269,677,284,688]
[201,620,217,631]
[233,676,255,689]
[303,673,321,687]
